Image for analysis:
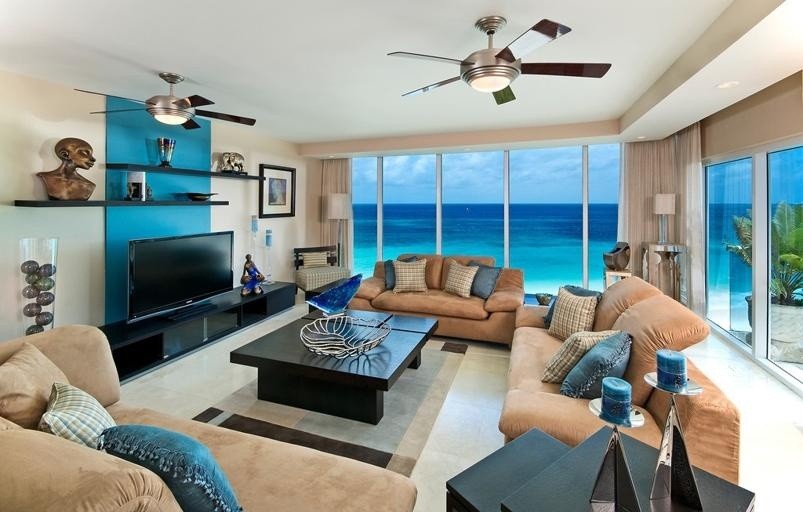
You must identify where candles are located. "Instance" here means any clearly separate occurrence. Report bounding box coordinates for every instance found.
[655,349,689,392]
[598,376,632,425]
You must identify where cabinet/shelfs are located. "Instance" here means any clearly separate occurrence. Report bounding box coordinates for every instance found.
[12,154,262,208]
[96,279,298,388]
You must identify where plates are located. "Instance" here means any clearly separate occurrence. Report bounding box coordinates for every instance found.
[644,372,704,395]
[589,397,645,427]
[300,315,391,359]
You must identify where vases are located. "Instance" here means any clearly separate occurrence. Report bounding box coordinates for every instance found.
[17,236,59,336]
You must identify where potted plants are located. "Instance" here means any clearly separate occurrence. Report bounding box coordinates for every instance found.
[723,198,803,364]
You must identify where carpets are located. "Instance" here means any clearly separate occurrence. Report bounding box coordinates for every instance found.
[191,339,468,478]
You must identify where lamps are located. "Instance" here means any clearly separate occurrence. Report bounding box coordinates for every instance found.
[461,66,519,95]
[146,108,194,126]
[325,192,354,268]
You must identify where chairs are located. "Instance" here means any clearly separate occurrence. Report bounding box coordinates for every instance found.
[294,245,337,296]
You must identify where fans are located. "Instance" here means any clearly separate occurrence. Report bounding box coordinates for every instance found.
[386,16,612,107]
[72,72,256,134]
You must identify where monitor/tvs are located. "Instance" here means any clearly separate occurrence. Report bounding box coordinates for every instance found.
[127,231,234,324]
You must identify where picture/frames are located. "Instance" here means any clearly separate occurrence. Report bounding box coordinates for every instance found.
[258,163,296,219]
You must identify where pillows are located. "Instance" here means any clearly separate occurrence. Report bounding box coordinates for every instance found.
[559,332,632,399]
[100,424,242,512]
[36,381,117,447]
[466,260,501,300]
[445,258,479,298]
[541,329,621,385]
[543,284,602,329]
[392,258,428,293]
[0,340,70,429]
[548,286,597,341]
[384,256,416,287]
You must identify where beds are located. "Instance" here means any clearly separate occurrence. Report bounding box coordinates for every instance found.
[641,192,686,253]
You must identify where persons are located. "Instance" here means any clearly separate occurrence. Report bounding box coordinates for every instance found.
[240,254,264,296]
[36,138,97,202]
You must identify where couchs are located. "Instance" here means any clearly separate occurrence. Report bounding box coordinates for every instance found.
[0,323,419,512]
[499,275,740,488]
[345,253,526,350]
